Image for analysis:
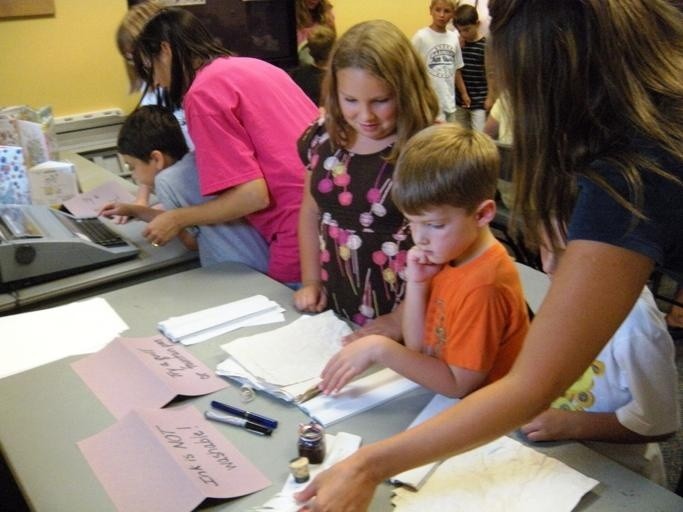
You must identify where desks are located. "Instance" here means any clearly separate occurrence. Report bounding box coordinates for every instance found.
[0,256,683,511]
[1,140,204,312]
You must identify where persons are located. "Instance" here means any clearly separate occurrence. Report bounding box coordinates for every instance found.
[255,2,337,110]
[516,208,679,493]
[318,121,532,401]
[117,2,195,224]
[412,2,471,120]
[451,4,496,137]
[133,8,321,294]
[486,89,516,210]
[97,106,270,275]
[287,1,680,511]
[290,17,444,328]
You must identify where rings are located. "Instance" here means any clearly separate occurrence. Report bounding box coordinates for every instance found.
[152,242,158,248]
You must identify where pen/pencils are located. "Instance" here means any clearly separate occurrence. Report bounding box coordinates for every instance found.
[203,400,278,436]
